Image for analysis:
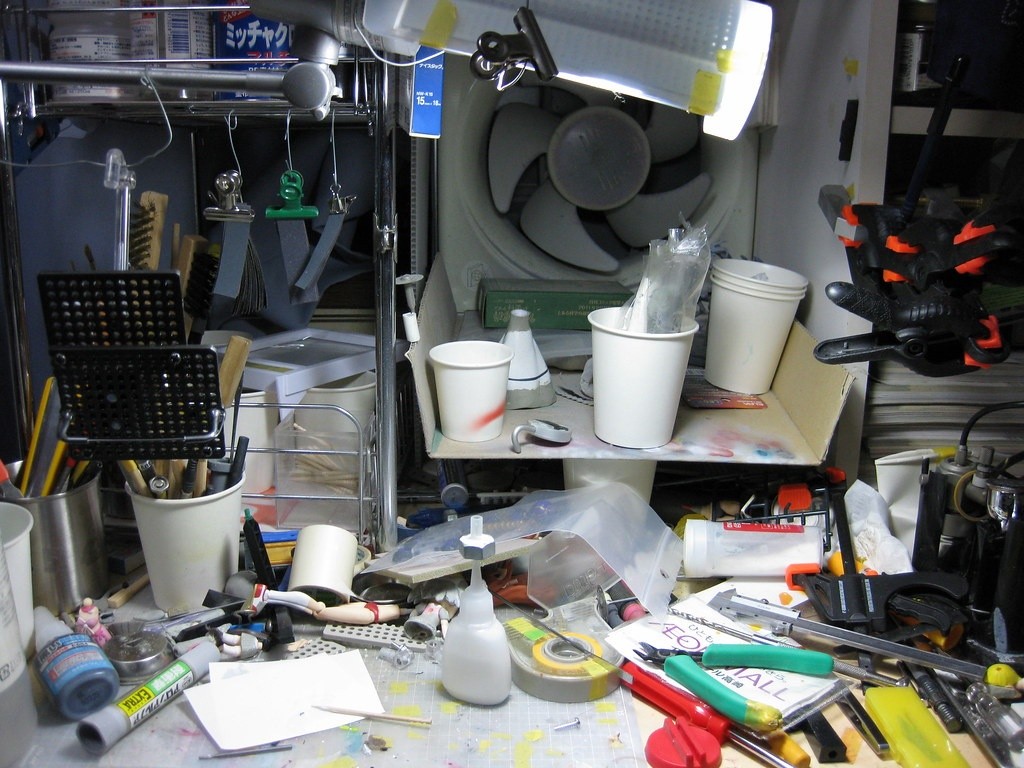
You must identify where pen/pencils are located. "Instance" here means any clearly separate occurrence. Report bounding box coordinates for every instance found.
[15,375,104,497]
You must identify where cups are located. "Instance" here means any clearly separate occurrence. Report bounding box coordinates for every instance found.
[123,472,248,615]
[0,501,36,663]
[223,388,282,493]
[0,459,110,615]
[429,339,515,444]
[875,448,972,563]
[703,258,809,395]
[293,368,377,475]
[587,307,700,449]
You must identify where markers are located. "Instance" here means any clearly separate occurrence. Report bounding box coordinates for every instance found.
[116,433,250,499]
[74,640,222,757]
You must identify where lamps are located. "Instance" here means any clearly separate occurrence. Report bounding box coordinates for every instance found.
[360,0,775,142]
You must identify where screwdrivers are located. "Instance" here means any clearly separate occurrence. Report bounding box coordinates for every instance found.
[928,640,1024,751]
[619,660,800,768]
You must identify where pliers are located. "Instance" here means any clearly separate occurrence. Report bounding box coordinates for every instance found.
[631,641,834,733]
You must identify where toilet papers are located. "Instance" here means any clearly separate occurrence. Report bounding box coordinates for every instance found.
[286,524,358,605]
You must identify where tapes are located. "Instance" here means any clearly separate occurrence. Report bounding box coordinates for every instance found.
[530,630,603,676]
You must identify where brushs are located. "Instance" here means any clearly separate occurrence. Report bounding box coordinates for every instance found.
[126,189,221,344]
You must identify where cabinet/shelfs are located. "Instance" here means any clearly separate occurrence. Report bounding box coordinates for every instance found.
[757,0,1024,499]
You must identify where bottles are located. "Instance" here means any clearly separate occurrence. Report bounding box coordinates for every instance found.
[0,540,37,768]
[33,603,122,720]
[439,514,513,706]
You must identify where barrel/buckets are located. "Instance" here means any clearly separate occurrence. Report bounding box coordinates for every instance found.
[47,0,132,101]
[130,0,212,73]
